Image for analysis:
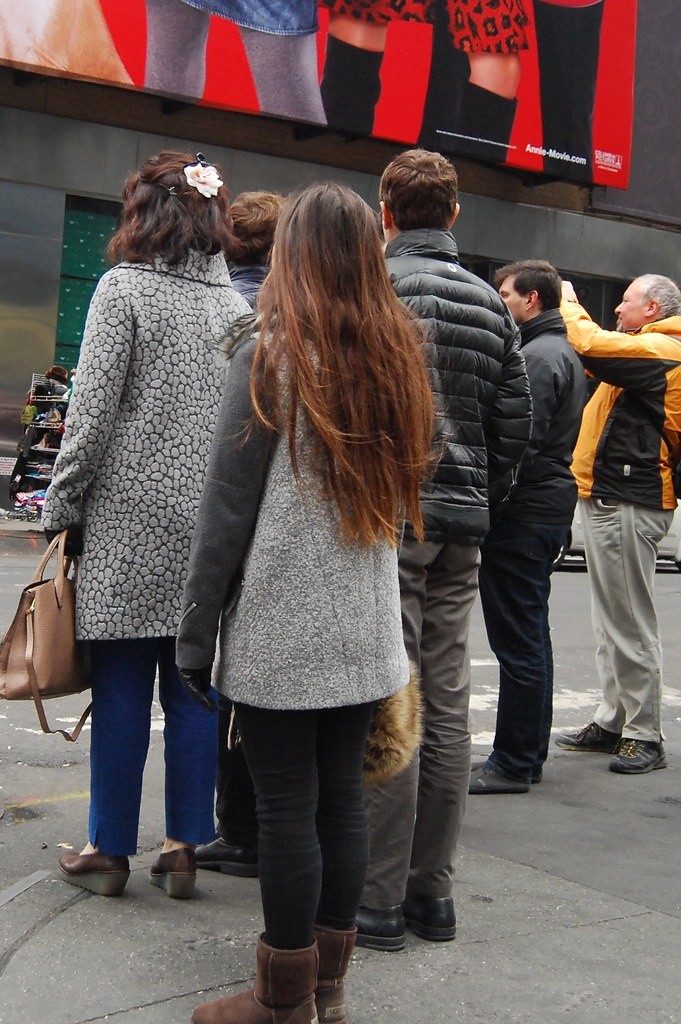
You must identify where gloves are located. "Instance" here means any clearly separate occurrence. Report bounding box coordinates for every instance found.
[180,668,218,713]
[45,527,84,557]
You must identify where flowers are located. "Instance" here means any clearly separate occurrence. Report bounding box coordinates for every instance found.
[182,152,223,197]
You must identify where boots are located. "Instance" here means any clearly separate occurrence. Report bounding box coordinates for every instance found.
[192,932,319,1024]
[315,924,357,1024]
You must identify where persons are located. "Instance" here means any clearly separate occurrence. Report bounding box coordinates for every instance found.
[320,0,530,164]
[39,153,255,902]
[356,147,532,954]
[558,275,681,772]
[195,190,285,879]
[467,261,587,795]
[143,0,328,125]
[175,181,433,1024]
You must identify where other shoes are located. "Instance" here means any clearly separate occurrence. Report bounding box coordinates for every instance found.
[468,770,529,794]
[471,762,543,783]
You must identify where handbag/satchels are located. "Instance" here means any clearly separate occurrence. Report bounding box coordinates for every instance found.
[672,459,681,499]
[0,529,93,700]
[360,659,421,782]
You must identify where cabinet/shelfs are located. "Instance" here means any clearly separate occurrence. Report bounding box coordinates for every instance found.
[8,372,69,521]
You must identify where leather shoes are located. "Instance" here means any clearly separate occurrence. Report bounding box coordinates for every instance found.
[195,838,258,877]
[402,896,457,940]
[354,904,406,951]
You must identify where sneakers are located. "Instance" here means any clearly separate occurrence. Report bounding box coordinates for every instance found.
[555,722,620,754]
[609,739,667,773]
[14,500,37,511]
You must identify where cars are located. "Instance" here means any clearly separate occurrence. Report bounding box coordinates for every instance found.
[552,498,681,571]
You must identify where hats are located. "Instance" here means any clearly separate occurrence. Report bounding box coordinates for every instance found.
[16,366,68,452]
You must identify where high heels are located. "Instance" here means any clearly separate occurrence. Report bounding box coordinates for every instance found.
[59,851,130,896]
[148,848,197,899]
